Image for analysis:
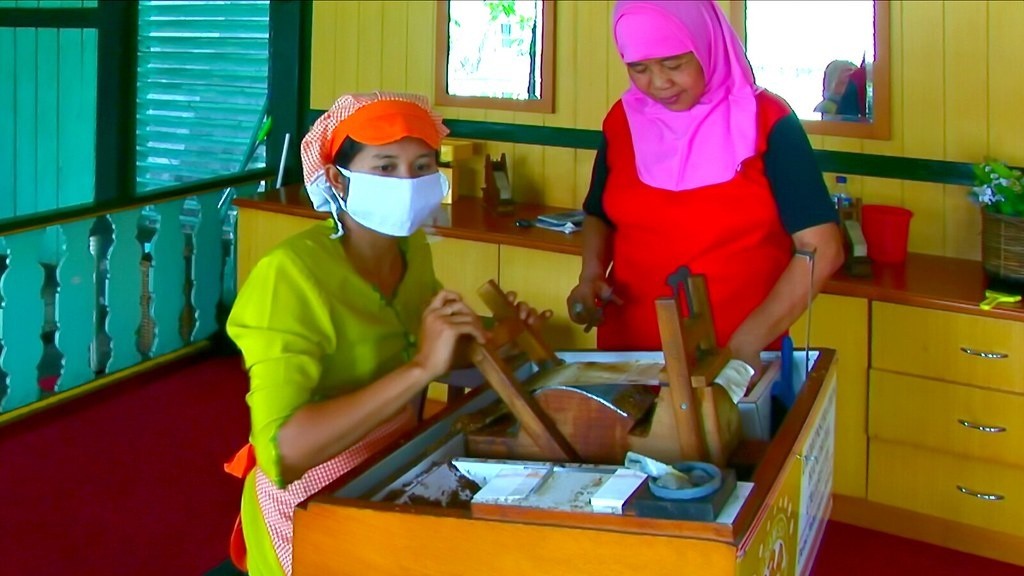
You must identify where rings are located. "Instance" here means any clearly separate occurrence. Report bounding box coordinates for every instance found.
[441,304,454,316]
[571,302,583,317]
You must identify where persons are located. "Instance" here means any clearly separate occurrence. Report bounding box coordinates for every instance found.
[226,88,556,576]
[564,0,849,392]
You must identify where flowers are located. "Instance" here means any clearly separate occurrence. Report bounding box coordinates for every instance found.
[968,158,1024,217]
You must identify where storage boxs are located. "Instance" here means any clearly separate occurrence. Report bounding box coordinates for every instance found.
[290,341,834,575]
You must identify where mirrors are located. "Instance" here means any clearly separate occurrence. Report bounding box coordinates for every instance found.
[435,1,554,112]
[728,1,891,142]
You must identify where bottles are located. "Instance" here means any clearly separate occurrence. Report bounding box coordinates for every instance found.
[830,176,852,212]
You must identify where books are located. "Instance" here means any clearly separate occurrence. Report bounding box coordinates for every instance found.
[530,211,584,234]
[404,462,738,517]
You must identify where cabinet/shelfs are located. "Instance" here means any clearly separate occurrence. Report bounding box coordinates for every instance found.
[233,193,1024,567]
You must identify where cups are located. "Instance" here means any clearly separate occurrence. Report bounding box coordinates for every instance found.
[861,204,913,262]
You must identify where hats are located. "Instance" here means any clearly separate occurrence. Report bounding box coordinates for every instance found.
[295,89,451,210]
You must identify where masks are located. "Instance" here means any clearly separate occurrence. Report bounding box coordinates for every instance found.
[327,163,451,237]
